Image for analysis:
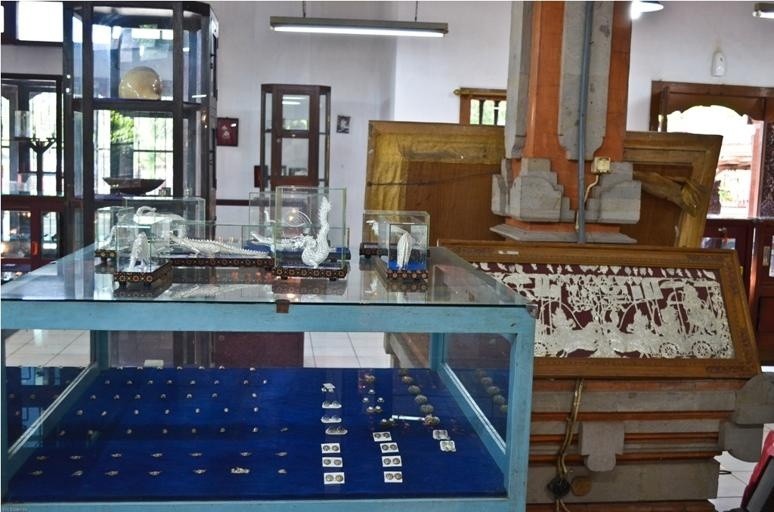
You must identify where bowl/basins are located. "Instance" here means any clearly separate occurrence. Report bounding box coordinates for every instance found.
[1,240,21,256]
[103,175,165,195]
[19,241,31,257]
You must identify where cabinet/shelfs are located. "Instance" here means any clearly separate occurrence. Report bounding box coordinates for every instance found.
[1,244,535,512]
[63,3,219,253]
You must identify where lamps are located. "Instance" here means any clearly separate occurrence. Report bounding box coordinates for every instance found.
[269,2,449,39]
